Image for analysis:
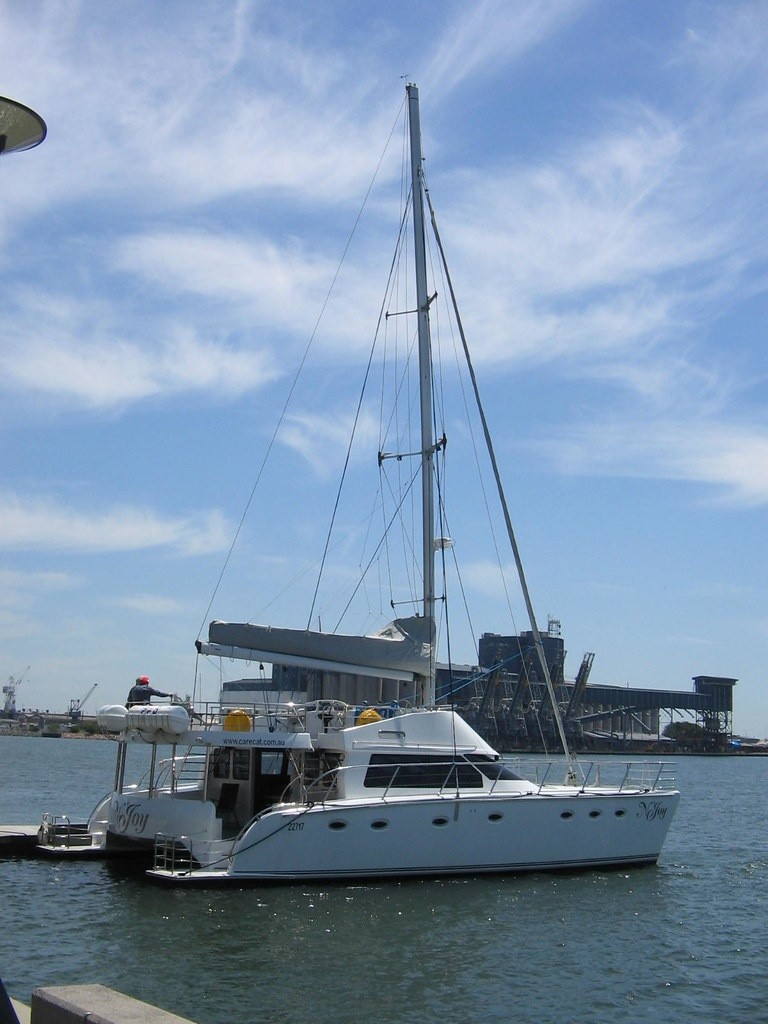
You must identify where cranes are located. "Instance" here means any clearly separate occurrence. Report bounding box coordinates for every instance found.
[2,666,31,715]
[68,682,99,720]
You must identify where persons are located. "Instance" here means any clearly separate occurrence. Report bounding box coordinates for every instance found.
[124,675,174,711]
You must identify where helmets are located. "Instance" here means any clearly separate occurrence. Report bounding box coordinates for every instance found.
[137,676,149,684]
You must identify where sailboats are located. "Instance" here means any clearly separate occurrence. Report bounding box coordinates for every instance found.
[85,84,682,892]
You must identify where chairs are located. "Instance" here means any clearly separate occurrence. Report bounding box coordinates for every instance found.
[215,783,241,830]
